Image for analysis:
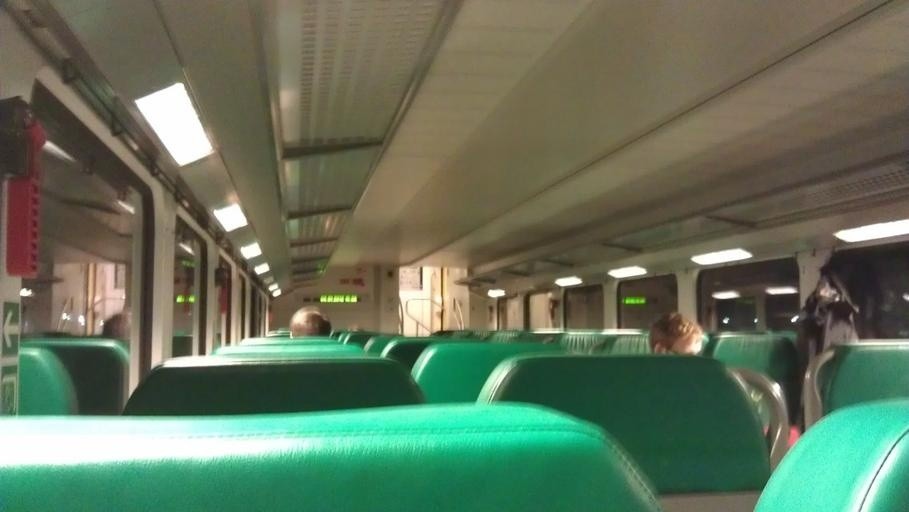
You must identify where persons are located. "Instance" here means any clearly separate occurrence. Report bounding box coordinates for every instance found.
[289,306,332,339]
[103,310,129,341]
[649,311,707,356]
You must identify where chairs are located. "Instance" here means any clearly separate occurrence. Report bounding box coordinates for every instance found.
[0,323,909,512]
